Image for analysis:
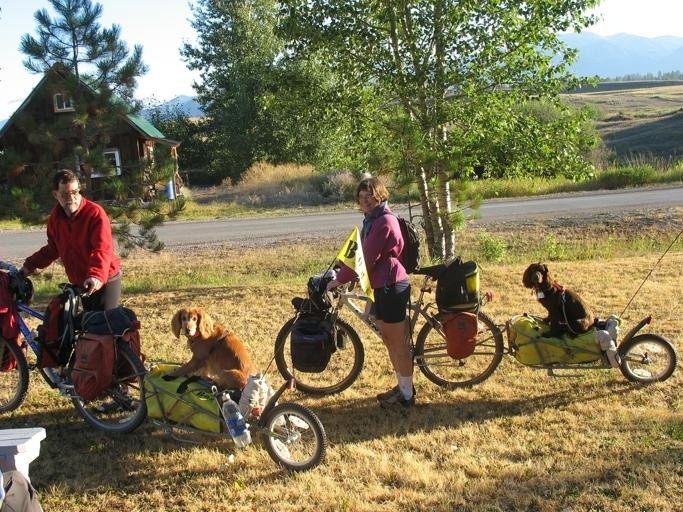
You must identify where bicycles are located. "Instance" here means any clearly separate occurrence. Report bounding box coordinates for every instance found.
[0,261,149,436]
[274,256,504,395]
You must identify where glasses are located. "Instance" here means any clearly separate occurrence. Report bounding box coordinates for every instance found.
[57,188,81,196]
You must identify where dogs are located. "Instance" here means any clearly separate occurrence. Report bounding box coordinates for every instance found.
[522,261,596,340]
[161,307,259,393]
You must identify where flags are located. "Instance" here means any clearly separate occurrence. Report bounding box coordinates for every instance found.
[337,227,375,304]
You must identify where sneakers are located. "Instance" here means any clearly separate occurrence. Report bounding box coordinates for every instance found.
[376,386,416,409]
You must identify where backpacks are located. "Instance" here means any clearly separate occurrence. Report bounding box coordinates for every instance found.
[290,315,347,373]
[37,292,146,400]
[143,363,222,433]
[508,314,601,365]
[376,211,420,274]
[444,312,478,359]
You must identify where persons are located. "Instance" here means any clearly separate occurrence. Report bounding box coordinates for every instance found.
[326,179,416,407]
[20,168,133,413]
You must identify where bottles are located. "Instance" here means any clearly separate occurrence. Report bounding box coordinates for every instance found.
[221,393,252,448]
